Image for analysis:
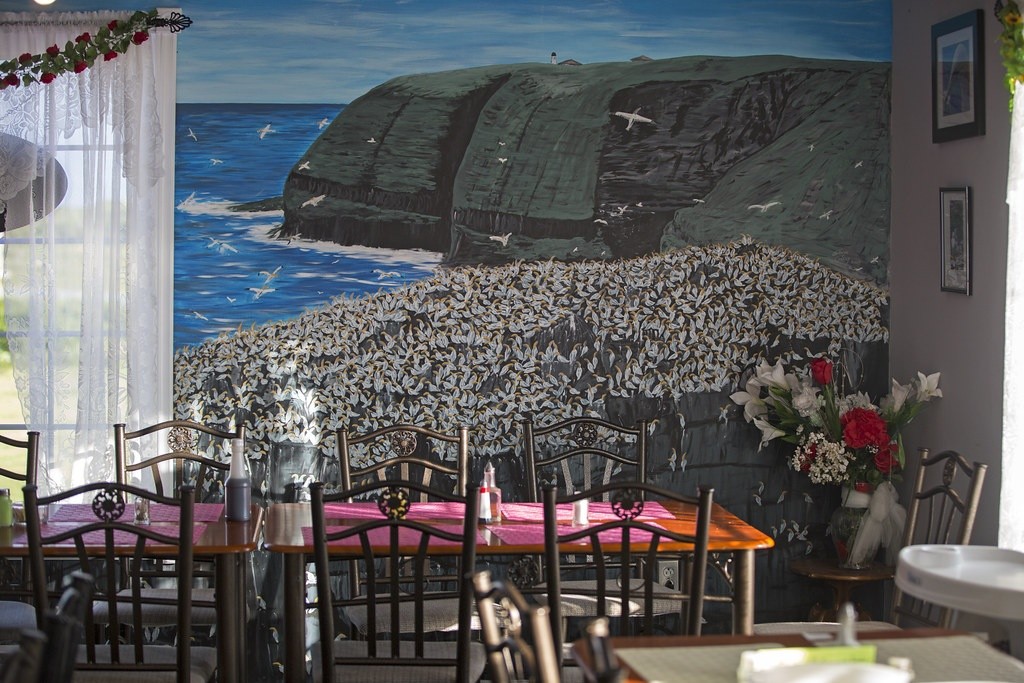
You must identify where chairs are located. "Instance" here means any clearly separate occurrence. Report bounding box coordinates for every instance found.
[0,418,717,683]
[786,446,988,629]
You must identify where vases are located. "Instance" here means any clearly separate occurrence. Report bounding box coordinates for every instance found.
[829,479,879,569]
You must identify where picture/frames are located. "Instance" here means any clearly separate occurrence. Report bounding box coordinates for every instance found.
[930,8,988,144]
[939,186,971,296]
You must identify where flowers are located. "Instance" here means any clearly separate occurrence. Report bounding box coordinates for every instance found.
[0,7,161,91]
[724,337,942,544]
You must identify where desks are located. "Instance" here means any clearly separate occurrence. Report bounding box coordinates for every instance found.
[1,501,268,683]
[258,497,775,683]
[570,627,1023,682]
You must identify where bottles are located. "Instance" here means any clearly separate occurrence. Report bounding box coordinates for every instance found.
[224,438,252,522]
[0,488,13,527]
[478,462,502,525]
[134,488,152,526]
[573,491,589,527]
